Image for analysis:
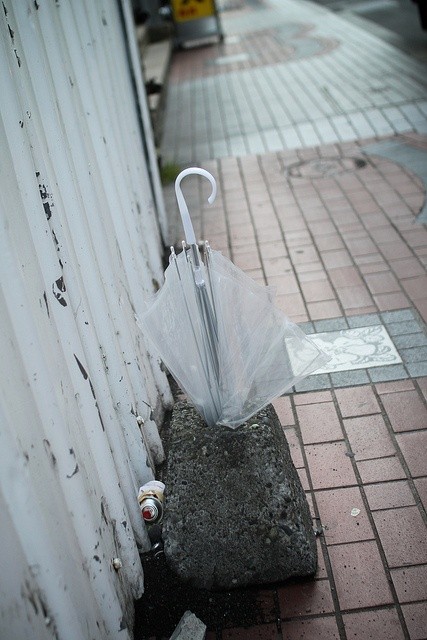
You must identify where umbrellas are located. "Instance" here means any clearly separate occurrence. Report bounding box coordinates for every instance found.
[134,168,332,429]
